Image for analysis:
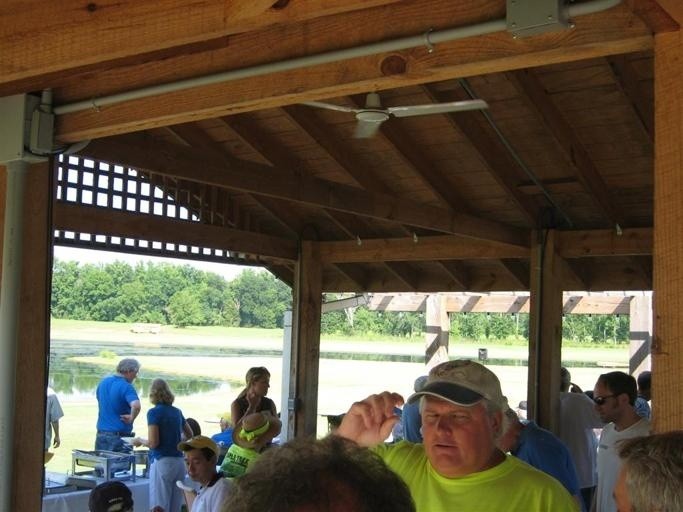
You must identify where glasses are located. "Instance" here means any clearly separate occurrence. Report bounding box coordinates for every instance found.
[594,395,615,405]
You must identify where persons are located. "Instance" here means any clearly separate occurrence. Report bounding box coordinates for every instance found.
[178,435,232,512]
[44,386,64,449]
[93,359,141,454]
[186,417,201,436]
[230,367,276,429]
[219,436,418,511]
[335,361,683,511]
[219,411,282,476]
[131,379,194,512]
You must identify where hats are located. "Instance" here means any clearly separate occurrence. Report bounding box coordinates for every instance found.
[232,411,282,448]
[178,435,220,463]
[408,361,503,406]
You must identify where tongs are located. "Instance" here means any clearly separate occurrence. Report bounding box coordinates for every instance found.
[72,449,96,456]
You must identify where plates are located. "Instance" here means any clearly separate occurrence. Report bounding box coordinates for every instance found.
[121,437,139,443]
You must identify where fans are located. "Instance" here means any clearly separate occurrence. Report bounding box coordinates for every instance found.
[299,93,489,142]
